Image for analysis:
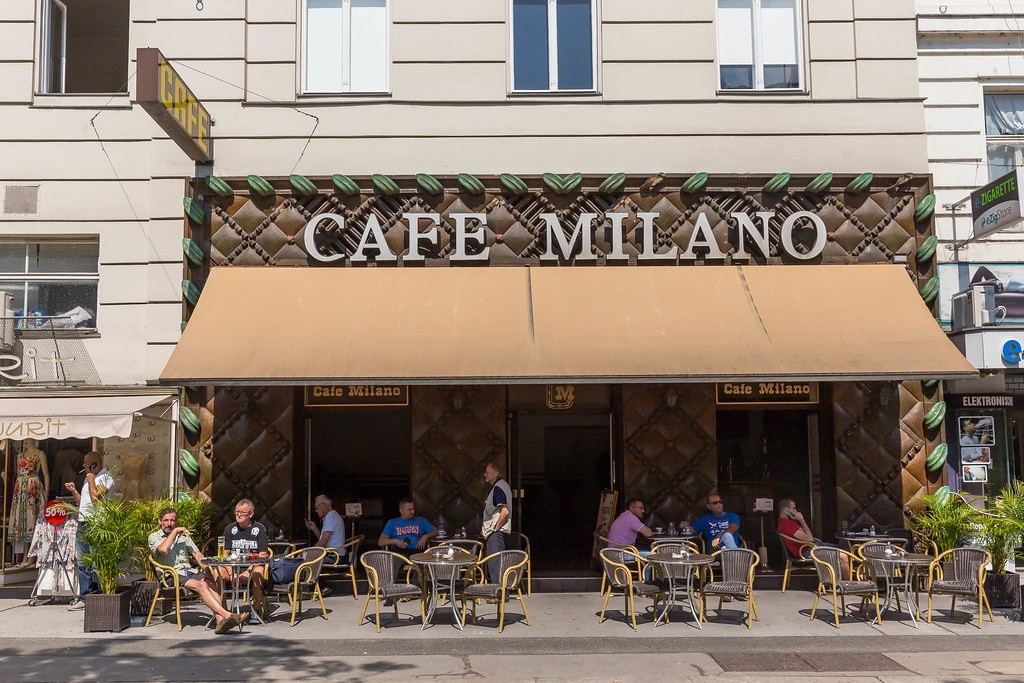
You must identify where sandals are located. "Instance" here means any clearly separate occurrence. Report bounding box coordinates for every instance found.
[215,616,238,633]
[231,612,250,624]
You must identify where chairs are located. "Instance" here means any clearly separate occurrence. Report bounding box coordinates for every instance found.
[145,529,995,633]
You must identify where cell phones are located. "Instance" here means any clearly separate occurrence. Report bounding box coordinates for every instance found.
[89,462,98,470]
[784,507,794,518]
[305,519,311,527]
[404,537,410,544]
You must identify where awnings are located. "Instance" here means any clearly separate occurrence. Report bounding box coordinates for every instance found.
[158,267,977,386]
[0,396,179,440]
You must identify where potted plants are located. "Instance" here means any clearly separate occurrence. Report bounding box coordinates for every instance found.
[904,479,1024,608]
[50,485,221,633]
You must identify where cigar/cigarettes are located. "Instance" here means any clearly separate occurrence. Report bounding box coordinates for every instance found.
[79,469,84,473]
[189,533,193,535]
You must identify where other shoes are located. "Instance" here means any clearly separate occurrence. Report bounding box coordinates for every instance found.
[67,600,85,610]
[487,598,509,603]
[248,608,262,623]
[321,587,333,596]
[724,595,734,602]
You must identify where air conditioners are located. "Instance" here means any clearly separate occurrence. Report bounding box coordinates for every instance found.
[951,285,995,331]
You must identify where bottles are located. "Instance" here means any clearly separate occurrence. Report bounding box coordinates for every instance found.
[668,521,676,535]
[870,526,875,535]
[884,541,893,554]
[461,527,466,537]
[17,308,43,328]
[218,536,259,562]
[678,543,687,557]
[279,530,284,537]
[448,544,454,561]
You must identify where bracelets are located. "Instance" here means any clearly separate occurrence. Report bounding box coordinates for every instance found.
[494,529,497,531]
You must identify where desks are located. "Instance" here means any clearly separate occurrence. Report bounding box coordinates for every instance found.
[409,553,478,631]
[863,552,935,629]
[429,535,482,554]
[834,533,890,552]
[646,531,699,543]
[203,557,270,633]
[647,551,715,631]
[267,539,306,555]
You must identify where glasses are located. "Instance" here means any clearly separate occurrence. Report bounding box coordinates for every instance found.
[631,506,645,510]
[234,510,251,515]
[315,503,324,510]
[707,500,723,505]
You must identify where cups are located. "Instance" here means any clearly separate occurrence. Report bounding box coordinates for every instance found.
[682,527,690,532]
[655,528,663,533]
[863,529,868,532]
[437,531,446,536]
[432,549,443,558]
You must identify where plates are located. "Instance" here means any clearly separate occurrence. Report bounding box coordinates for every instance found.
[860,532,869,535]
[680,532,693,535]
[275,537,284,540]
[436,536,448,539]
[653,532,666,534]
[453,536,468,538]
[869,535,888,536]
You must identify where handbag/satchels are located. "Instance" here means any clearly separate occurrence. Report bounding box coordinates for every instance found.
[260,557,306,596]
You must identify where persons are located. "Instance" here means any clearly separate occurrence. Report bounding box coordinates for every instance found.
[609,500,652,580]
[961,422,988,444]
[481,463,512,603]
[378,502,439,602]
[982,448,989,462]
[306,495,346,600]
[964,467,974,480]
[65,451,116,611]
[778,497,865,580]
[0,438,49,568]
[679,495,740,601]
[214,499,269,624]
[148,508,249,633]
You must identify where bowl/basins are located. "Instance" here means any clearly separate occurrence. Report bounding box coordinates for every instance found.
[454,534,461,536]
